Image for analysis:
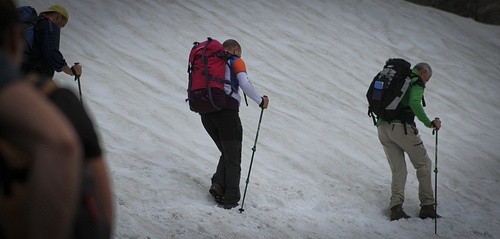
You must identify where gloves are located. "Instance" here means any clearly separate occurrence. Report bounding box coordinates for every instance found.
[71,65,82,79]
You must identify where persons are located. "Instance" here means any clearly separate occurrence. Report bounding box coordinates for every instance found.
[376,62,442,222]
[199,39,269,208]
[0,0,118,239]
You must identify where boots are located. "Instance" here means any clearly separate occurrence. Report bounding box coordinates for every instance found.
[391,205,409,221]
[419,204,442,219]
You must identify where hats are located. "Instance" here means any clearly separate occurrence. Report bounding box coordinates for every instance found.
[38,5,70,22]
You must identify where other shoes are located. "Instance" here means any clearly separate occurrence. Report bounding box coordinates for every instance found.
[210,182,224,204]
[223,203,238,209]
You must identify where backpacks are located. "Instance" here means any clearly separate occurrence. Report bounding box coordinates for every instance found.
[18,6,46,52]
[367,59,425,118]
[187,39,237,111]
